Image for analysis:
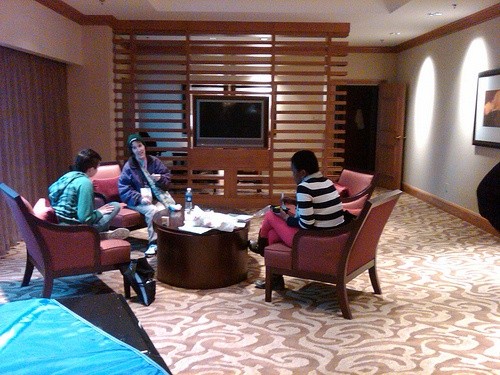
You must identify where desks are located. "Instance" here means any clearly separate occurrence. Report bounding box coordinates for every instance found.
[151,205,251,292]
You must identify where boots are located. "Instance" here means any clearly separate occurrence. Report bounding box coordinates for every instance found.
[248,234,267,256]
[255,272,285,290]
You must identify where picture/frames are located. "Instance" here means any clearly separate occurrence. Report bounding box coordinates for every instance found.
[472,70,500,148]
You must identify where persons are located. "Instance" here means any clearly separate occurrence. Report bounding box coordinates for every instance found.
[48,148,130,240]
[117,134,175,256]
[248,150,344,291]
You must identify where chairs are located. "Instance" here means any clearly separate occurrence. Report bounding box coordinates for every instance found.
[282,170,376,219]
[88,164,143,249]
[0,183,131,298]
[264,189,401,321]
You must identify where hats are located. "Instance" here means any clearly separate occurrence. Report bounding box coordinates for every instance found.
[127,134,149,146]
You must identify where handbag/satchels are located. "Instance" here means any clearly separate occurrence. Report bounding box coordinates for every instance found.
[121,255,156,306]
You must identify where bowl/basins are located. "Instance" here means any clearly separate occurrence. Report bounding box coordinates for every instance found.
[271,204,290,213]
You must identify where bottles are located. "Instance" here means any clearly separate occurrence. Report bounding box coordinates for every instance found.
[185,188,193,209]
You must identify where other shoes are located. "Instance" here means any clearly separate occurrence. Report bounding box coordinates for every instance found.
[99,227,130,241]
[144,243,157,253]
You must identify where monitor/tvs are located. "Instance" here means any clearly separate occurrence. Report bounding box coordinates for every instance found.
[196,100,264,147]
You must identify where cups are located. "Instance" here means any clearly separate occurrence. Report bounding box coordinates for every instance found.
[161,215,169,227]
